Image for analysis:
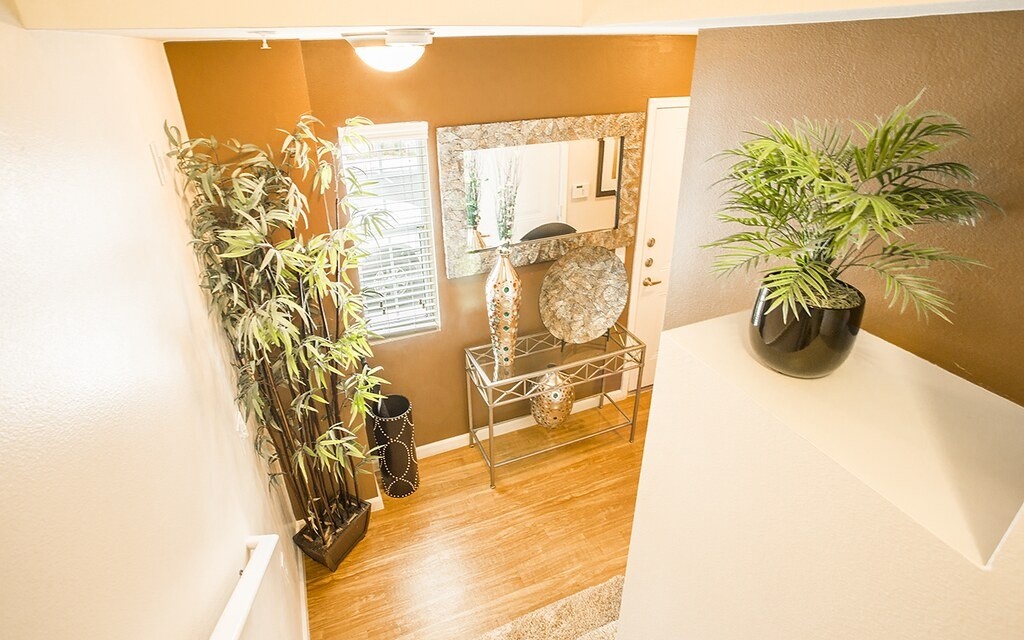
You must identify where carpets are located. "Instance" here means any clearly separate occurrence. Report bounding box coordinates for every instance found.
[478,574,624,640]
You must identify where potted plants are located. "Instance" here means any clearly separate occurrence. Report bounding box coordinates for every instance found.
[162,113,396,574]
[693,86,1009,378]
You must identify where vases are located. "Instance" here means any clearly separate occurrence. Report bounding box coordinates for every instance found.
[484,252,522,368]
[530,363,576,429]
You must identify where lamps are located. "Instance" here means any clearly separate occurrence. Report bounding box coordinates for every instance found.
[343,33,428,73]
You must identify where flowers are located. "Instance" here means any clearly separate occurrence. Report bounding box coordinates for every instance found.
[490,150,523,253]
[466,152,490,223]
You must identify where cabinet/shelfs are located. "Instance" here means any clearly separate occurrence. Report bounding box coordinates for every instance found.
[465,320,647,489]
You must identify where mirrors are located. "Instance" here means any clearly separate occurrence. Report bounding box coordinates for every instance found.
[436,111,647,278]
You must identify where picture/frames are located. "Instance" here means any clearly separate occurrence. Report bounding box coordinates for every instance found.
[596,137,621,199]
